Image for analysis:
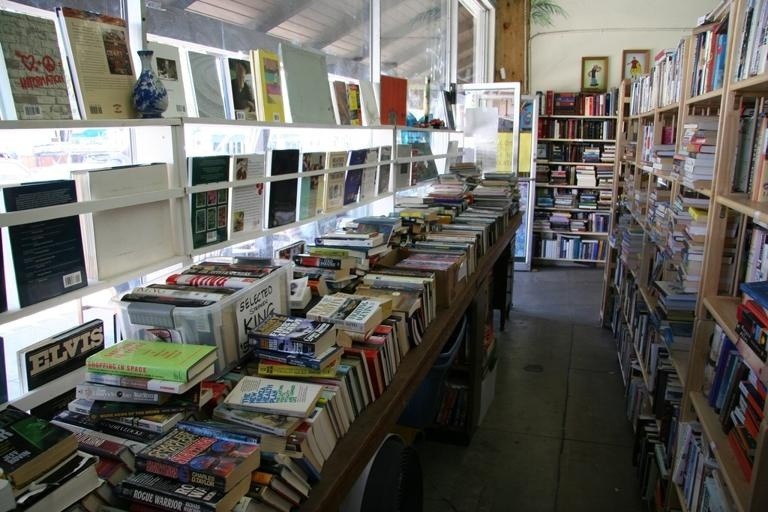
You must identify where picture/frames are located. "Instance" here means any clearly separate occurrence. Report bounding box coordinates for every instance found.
[581,49,652,94]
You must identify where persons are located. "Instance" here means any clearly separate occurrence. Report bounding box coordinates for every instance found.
[586,64,604,86]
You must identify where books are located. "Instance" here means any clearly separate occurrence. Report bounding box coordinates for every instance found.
[612,0,768,512]
[535,91,618,261]
[0,6,524,512]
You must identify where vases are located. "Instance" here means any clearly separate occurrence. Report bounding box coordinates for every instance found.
[130,50,169,120]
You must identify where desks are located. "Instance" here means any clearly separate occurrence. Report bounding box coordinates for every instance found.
[289,212,530,510]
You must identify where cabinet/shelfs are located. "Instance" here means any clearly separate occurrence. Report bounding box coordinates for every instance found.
[529,90,620,273]
[597,1,767,511]
[1,2,461,412]
